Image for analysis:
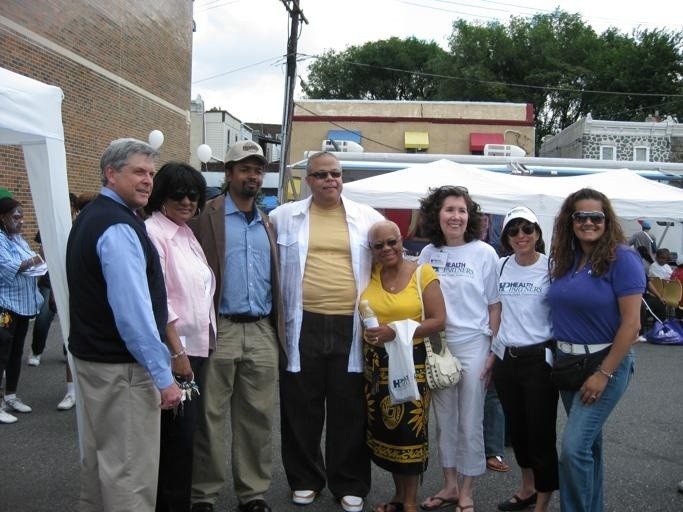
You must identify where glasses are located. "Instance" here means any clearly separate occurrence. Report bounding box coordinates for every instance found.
[507,222,535,237]
[373,239,399,250]
[167,190,201,202]
[571,210,606,223]
[308,171,342,179]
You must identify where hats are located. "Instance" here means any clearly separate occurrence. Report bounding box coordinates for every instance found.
[224,139,267,167]
[642,220,651,229]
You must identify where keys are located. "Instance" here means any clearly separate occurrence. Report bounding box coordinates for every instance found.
[172,370,201,402]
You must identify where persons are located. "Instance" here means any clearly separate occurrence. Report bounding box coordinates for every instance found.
[1,191,100,424]
[144,160,219,511]
[188,139,288,512]
[65,137,183,512]
[483,362,511,472]
[546,188,646,512]
[357,220,447,512]
[267,151,386,512]
[631,218,682,344]
[495,207,559,511]
[418,185,503,512]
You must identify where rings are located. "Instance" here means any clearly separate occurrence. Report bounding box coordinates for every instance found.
[590,395,597,401]
[168,404,173,407]
[375,336,379,343]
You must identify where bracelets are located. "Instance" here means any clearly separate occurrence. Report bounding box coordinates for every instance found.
[170,348,185,359]
[599,366,614,379]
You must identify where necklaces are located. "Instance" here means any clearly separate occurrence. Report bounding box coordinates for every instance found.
[390,287,396,291]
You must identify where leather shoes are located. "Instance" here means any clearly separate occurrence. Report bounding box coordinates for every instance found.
[191,502,214,512]
[238,499,272,512]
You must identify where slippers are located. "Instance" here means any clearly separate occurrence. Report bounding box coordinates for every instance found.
[371,500,404,512]
[455,499,475,512]
[419,493,458,511]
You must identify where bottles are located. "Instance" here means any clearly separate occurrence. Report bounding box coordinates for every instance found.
[359,300,380,345]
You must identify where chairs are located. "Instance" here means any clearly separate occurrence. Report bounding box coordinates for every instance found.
[647,276,683,321]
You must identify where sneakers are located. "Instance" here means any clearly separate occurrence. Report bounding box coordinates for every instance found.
[292,488,316,505]
[0,407,19,424]
[340,494,364,512]
[56,391,76,411]
[1,396,33,413]
[498,490,538,511]
[26,349,41,367]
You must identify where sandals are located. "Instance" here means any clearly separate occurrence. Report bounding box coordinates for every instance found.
[485,455,513,472]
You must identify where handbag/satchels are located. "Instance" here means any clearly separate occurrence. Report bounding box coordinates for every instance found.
[424,345,464,392]
[383,318,423,407]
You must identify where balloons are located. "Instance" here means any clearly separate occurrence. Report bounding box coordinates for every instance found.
[148,130,165,150]
[197,144,212,164]
